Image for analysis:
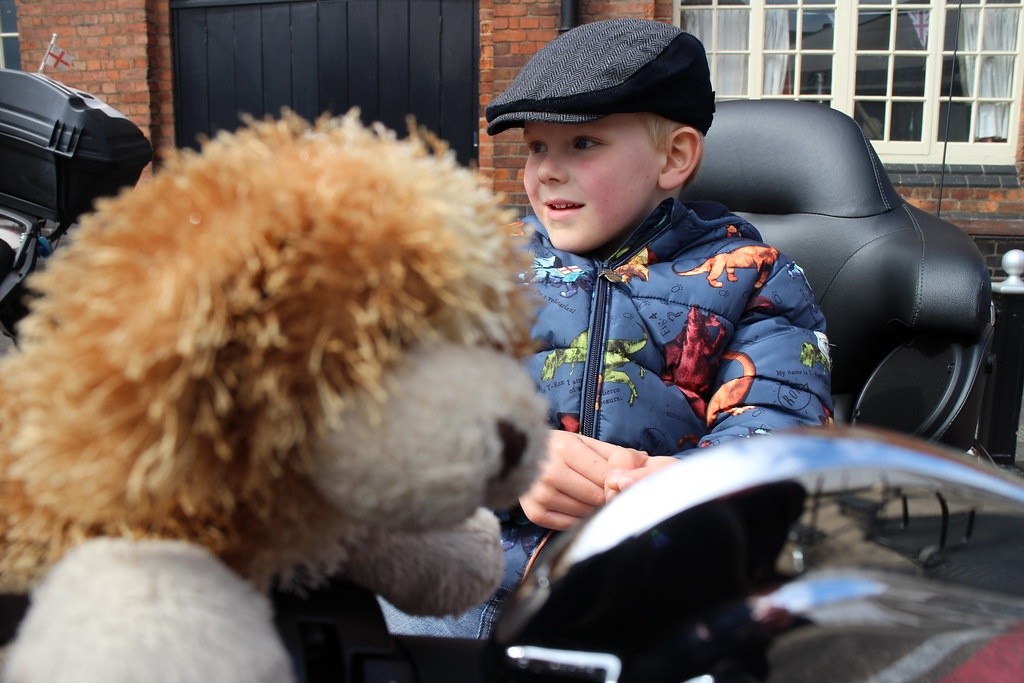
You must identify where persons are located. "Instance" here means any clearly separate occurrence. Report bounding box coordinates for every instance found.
[373,21,830,635]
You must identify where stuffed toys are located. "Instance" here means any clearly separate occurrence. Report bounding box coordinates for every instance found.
[1,104,551,683]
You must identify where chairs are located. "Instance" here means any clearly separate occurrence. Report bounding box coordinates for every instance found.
[681,101,994,449]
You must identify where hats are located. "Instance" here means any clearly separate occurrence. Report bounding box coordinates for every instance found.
[485,19,716,136]
